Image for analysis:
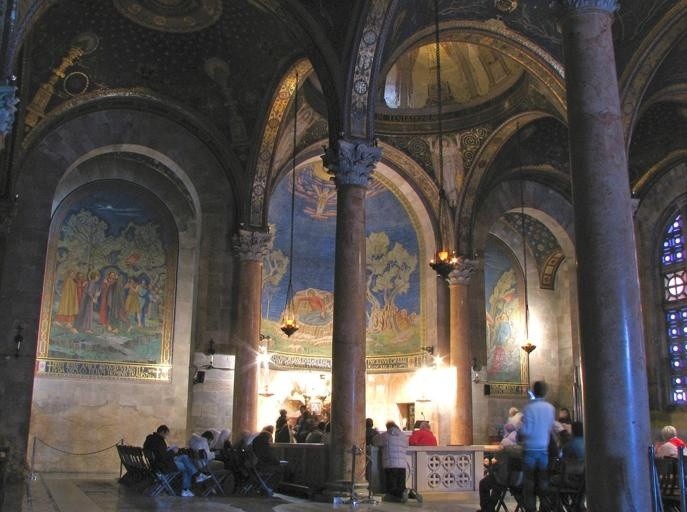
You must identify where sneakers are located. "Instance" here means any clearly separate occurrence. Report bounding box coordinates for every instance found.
[182,489,194,496]
[410,489,422,503]
[196,473,211,483]
[402,488,409,502]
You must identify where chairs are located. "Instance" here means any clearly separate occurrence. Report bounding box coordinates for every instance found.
[116,443,274,500]
[497,457,686,512]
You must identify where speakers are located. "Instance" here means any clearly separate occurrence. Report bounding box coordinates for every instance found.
[484,385,491,395]
[197,371,204,383]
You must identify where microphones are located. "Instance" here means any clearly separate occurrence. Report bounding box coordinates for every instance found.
[421,412,425,421]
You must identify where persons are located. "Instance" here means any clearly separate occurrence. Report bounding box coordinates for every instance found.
[428,134,462,208]
[56,270,148,335]
[298,289,331,325]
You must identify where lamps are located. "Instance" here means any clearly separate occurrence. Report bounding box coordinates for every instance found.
[430,2,460,284]
[279,69,301,339]
[516,119,539,355]
